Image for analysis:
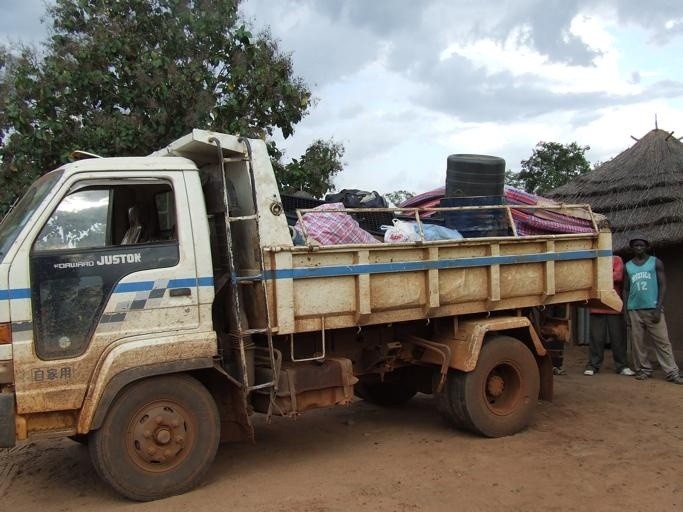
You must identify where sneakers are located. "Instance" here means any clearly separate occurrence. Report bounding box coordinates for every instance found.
[619,367,636,376]
[584,370,594,376]
[635,374,648,380]
[553,366,566,375]
[668,376,683,384]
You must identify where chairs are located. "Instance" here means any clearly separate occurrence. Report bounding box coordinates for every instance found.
[119,204,150,245]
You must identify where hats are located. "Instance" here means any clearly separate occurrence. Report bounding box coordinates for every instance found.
[629,232,649,248]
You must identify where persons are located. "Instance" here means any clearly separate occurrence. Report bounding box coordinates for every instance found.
[584,256,635,376]
[623,236,683,384]
[549,340,567,375]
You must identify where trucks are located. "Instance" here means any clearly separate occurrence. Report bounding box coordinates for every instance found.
[0,128,624,500]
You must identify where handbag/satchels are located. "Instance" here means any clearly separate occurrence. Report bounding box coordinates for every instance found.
[294,202,382,246]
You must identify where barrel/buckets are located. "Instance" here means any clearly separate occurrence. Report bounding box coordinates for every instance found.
[445,153,505,198]
